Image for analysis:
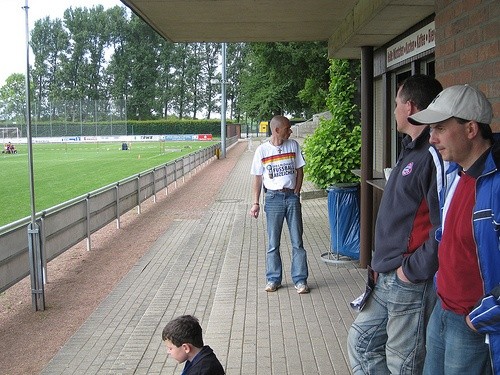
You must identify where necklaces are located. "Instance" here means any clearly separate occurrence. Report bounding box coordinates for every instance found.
[273,142,282,154]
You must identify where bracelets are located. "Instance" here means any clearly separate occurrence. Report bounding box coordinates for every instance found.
[254,203,258,205]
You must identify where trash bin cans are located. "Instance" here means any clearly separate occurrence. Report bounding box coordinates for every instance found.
[319,181,361,266]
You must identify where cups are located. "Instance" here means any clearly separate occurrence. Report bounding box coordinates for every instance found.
[384,167,393,182]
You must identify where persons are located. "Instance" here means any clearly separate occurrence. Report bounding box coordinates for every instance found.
[406,83,500,375]
[162,315,225,375]
[251,115,310,294]
[347,72,449,375]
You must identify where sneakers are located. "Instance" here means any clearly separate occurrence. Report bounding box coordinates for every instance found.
[295,282,310,294]
[265,281,282,292]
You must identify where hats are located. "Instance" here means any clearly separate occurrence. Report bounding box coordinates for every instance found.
[407,84,493,126]
